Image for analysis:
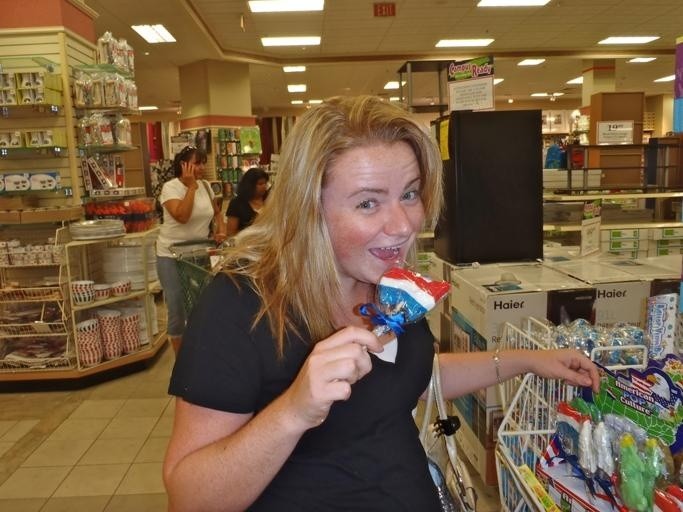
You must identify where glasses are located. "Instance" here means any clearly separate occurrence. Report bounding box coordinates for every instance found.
[178,145,198,157]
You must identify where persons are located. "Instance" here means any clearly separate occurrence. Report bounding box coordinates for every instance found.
[157,145,226,361]
[221,166,268,236]
[157,93,602,511]
[544,137,563,170]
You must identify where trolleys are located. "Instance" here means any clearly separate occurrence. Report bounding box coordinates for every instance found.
[168,238,222,322]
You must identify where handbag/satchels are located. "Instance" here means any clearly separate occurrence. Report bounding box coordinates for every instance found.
[419,352,478,512]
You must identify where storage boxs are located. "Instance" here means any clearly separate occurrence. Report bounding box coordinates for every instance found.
[413,251,683,512]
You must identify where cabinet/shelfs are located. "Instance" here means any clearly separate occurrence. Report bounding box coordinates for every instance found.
[169,123,261,206]
[2,64,169,378]
[398,60,450,119]
[542,95,683,257]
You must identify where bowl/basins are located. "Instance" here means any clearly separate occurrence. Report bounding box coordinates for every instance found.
[71,279,132,306]
[76,308,141,368]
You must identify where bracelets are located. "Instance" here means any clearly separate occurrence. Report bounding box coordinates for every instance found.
[492,348,503,384]
